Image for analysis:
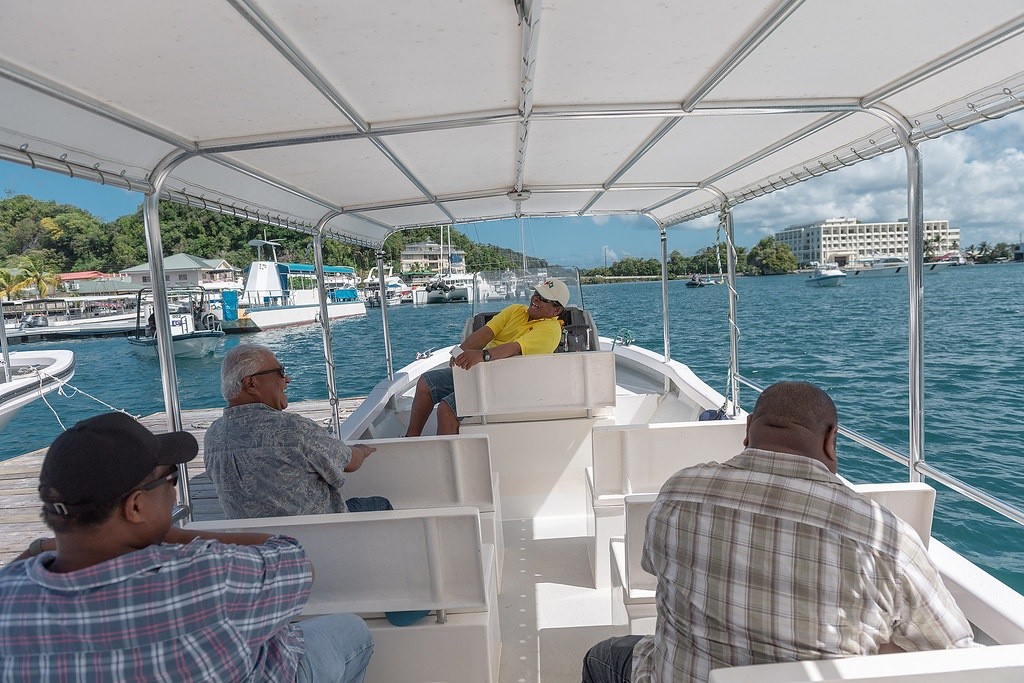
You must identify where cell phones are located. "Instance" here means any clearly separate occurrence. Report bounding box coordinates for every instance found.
[449,345,465,359]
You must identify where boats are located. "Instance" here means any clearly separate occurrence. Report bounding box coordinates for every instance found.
[126,284,226,360]
[840,255,958,278]
[685,280,705,287]
[703,279,723,285]
[0,302,76,432]
[943,253,967,265]
[994,257,1011,264]
[805,261,846,287]
[184,239,366,333]
[365,266,507,306]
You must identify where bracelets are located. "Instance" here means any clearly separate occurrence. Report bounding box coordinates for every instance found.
[30,537,48,555]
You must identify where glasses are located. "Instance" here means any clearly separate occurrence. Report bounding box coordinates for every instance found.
[241,367,285,383]
[534,289,559,307]
[107,464,179,507]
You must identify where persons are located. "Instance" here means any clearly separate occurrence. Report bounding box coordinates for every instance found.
[405,279,570,437]
[581,381,974,683]
[203,343,394,520]
[0,412,375,683]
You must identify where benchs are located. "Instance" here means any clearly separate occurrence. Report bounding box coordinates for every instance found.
[708,644,1024,683]
[584,420,747,589]
[181,506,502,683]
[609,483,934,634]
[451,350,617,518]
[339,433,503,595]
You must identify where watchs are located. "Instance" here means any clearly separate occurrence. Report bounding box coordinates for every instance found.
[483,350,491,362]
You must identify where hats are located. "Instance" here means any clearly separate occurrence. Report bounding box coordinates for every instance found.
[38,413,199,516]
[531,278,570,309]
